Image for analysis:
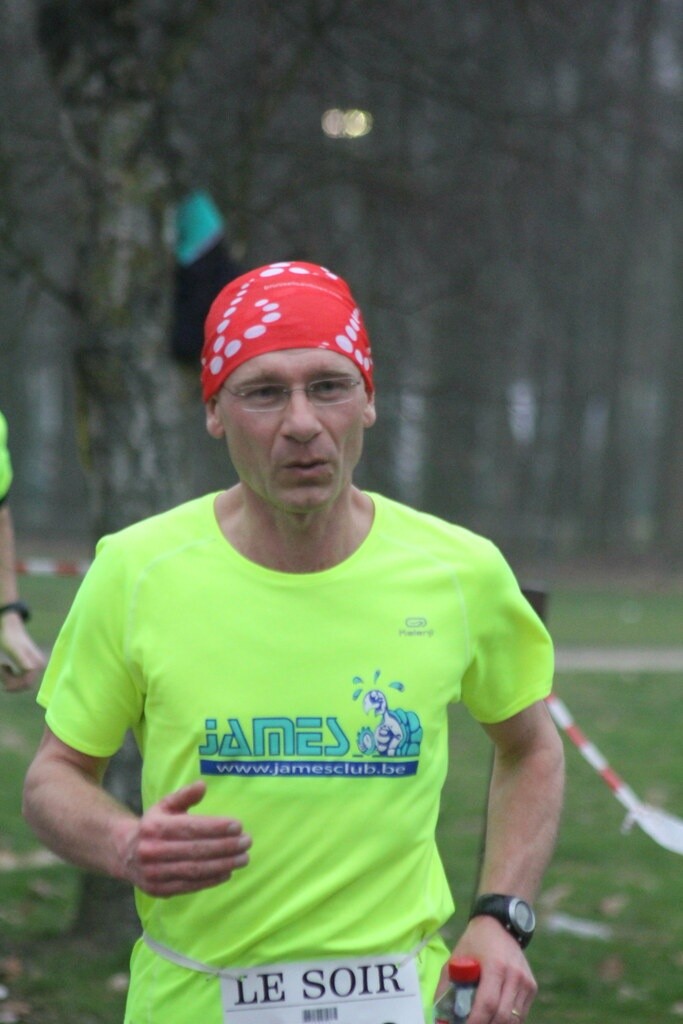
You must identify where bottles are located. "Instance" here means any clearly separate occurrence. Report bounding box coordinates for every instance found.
[432,956,481,1024]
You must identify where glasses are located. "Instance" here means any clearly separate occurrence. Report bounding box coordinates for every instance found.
[222,369,362,412]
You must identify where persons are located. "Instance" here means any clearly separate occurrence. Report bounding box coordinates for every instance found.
[22,263,565,1024]
[0,415,45,690]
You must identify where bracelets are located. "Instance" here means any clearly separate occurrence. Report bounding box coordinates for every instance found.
[0,603,30,620]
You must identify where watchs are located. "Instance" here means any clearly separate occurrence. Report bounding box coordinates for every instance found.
[469,894,536,949]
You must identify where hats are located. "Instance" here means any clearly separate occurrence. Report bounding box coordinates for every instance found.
[201,261,372,403]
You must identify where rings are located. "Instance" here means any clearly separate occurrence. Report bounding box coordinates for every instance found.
[511,1010,521,1016]
